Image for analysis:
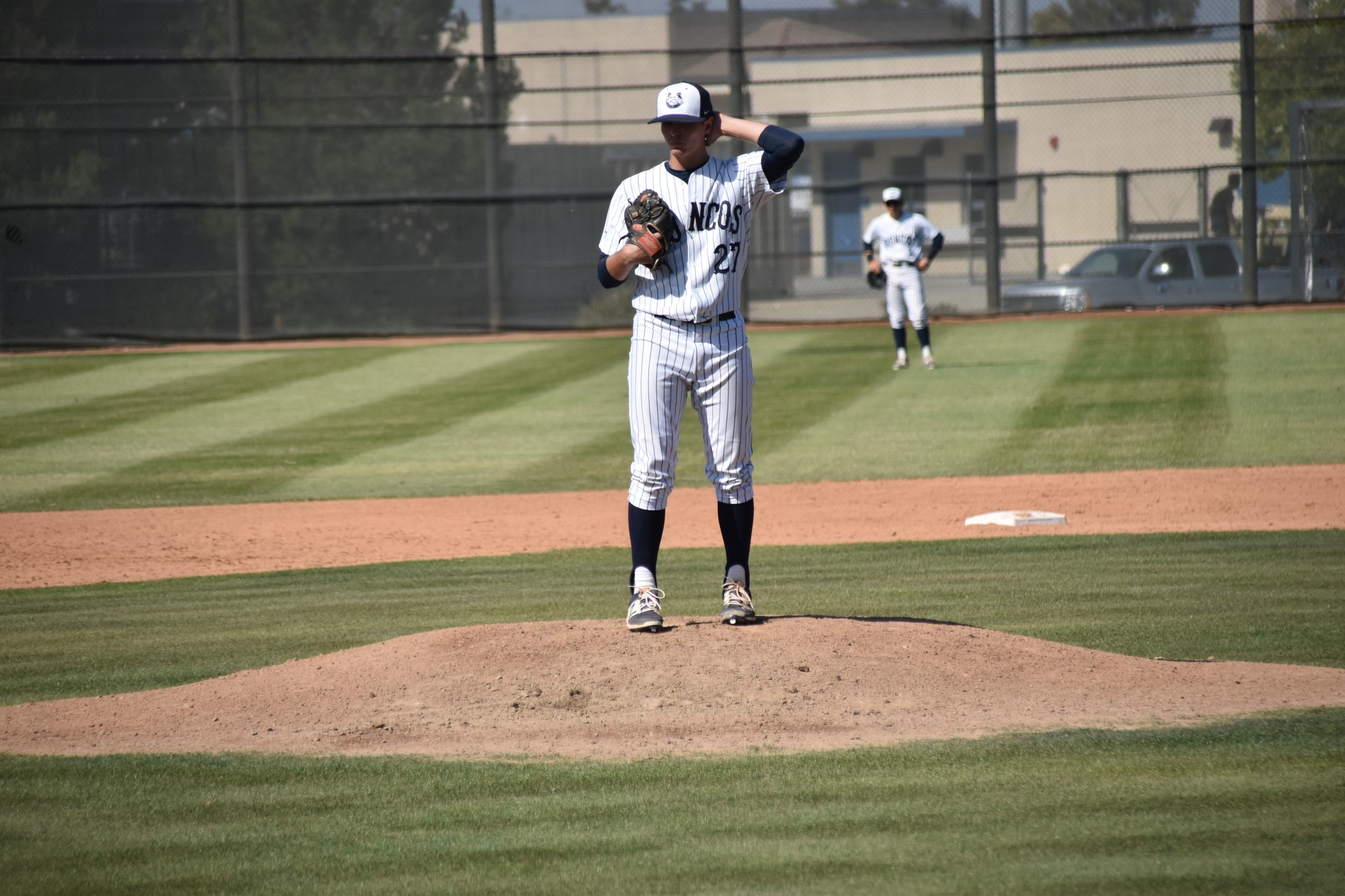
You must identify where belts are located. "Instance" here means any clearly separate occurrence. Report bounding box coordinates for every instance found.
[894,263,913,266]
[654,311,735,325]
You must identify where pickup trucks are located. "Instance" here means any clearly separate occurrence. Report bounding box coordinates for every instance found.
[1000,236,1341,313]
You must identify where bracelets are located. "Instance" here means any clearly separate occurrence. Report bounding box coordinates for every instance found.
[866,255,872,262]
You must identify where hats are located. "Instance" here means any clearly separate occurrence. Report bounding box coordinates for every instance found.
[882,187,901,203]
[647,81,712,123]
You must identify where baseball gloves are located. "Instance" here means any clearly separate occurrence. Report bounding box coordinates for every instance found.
[867,268,887,289]
[624,189,680,272]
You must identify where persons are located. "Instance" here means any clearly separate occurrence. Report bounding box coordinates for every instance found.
[598,82,807,633]
[862,187,945,371]
[1209,173,1240,236]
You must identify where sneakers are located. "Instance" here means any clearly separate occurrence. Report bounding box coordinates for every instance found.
[719,575,756,624]
[627,585,666,633]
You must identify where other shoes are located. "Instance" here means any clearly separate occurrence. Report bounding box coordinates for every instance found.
[894,360,908,371]
[925,358,935,369]
